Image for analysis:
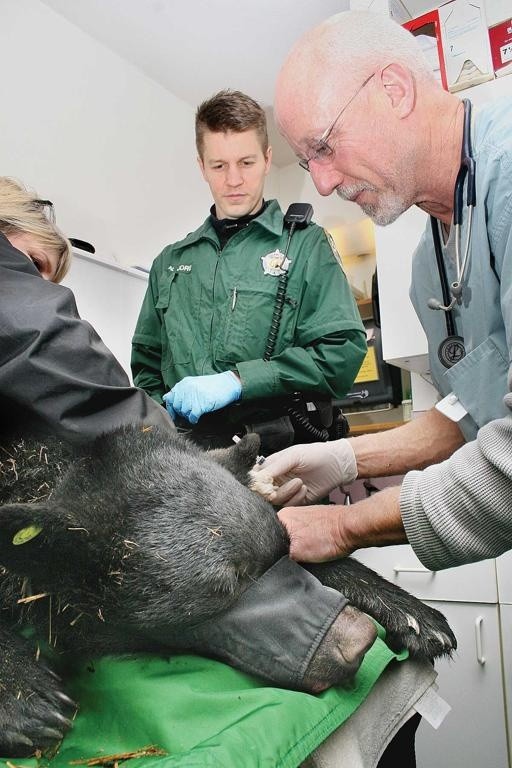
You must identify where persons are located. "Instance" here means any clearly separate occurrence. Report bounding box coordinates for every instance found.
[249,9,512,570]
[0,173,179,463]
[130,91,368,512]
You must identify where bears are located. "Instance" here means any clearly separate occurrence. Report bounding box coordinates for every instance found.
[0,396,456,755]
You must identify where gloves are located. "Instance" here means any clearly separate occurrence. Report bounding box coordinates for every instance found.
[161,369,242,424]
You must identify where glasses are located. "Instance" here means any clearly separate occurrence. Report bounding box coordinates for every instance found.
[299,73,376,172]
